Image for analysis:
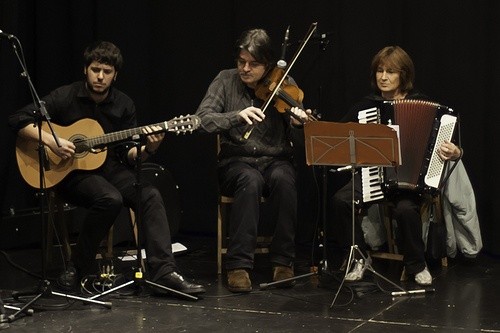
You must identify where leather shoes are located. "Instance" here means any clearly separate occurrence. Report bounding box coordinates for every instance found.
[75,241,99,278]
[150,262,207,294]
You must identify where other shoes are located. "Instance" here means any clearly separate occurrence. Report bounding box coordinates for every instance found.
[227,269,252,292]
[346,258,365,282]
[273,265,295,288]
[415,264,432,285]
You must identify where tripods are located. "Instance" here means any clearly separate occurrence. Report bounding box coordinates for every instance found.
[258,32,412,309]
[7,35,202,322]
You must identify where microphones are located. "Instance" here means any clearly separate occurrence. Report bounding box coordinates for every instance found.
[277,28,290,69]
[0,30,14,37]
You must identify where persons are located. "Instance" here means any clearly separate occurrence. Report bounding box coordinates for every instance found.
[334,44,463,285]
[9,41,204,297]
[195,28,312,292]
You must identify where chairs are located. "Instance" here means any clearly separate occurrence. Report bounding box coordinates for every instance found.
[217,135,271,274]
[44,186,148,287]
[344,190,448,282]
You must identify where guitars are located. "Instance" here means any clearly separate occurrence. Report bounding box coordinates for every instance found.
[15,114,202,191]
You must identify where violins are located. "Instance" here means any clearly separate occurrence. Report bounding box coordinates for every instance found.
[246,65,322,122]
